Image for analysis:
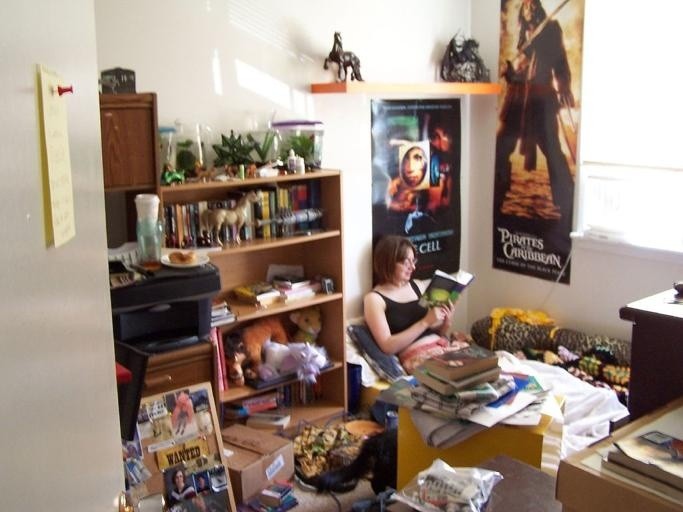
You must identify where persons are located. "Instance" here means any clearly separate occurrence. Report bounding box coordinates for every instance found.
[361,233,474,377]
[498,1,577,219]
[166,470,194,504]
[196,473,209,494]
[397,145,429,187]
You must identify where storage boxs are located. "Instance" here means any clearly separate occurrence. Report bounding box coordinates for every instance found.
[554,398,682,512]
[210,414,300,504]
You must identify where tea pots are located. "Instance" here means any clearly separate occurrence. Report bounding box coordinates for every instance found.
[170,116,211,172]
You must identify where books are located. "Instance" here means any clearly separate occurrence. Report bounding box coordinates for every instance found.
[212,277,322,325]
[419,269,472,309]
[409,342,533,429]
[222,373,325,423]
[160,178,323,250]
[244,480,297,511]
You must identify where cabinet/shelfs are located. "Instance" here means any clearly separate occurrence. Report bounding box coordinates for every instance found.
[157,156,350,438]
[97,92,161,200]
[126,339,214,404]
[612,282,682,420]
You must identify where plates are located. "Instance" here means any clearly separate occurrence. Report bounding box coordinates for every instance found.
[159,251,210,268]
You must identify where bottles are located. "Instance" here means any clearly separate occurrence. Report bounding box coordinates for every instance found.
[286,148,296,171]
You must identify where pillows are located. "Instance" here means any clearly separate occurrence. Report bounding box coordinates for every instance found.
[340,313,410,384]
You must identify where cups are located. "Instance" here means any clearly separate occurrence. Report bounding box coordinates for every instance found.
[134,217,163,263]
[134,194,160,224]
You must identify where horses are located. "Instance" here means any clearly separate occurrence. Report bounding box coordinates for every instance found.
[202,190,258,247]
[323,31,364,82]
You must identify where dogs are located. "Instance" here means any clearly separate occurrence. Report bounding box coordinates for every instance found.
[309,428,396,495]
